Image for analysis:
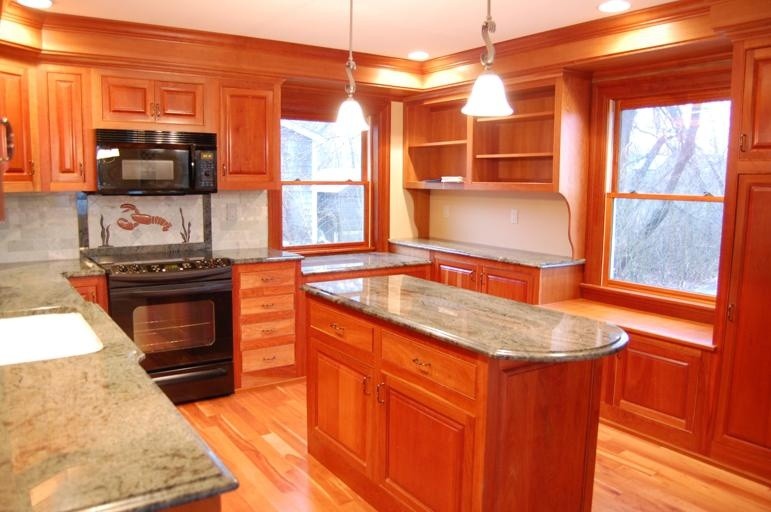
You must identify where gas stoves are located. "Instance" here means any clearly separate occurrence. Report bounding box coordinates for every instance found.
[89,248,231,277]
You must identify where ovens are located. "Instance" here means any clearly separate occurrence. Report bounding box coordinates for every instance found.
[109,279,234,404]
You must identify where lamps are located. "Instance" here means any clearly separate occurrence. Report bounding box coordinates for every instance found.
[333,1,372,132]
[461,0,514,119]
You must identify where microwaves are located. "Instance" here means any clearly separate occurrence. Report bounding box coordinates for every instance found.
[96,129,217,196]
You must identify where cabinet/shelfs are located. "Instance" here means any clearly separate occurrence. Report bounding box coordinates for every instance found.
[232,260,304,392]
[0,45,97,192]
[402,68,589,194]
[431,251,587,305]
[217,77,281,192]
[89,65,217,136]
[698,29,771,491]
[306,292,608,511]
